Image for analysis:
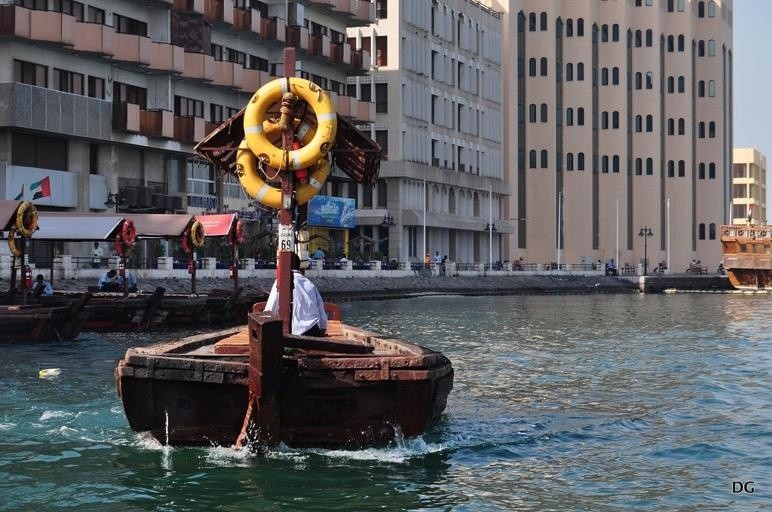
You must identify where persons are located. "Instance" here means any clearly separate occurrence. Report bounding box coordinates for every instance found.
[263,254,328,338]
[116,270,138,294]
[432,251,442,262]
[98,270,118,290]
[425,253,432,263]
[607,259,617,276]
[717,262,725,275]
[514,257,523,271]
[31,274,54,297]
[441,255,448,272]
[686,260,702,274]
[311,247,326,260]
[89,242,104,269]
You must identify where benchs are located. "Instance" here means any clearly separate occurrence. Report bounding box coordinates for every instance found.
[690,267,708,275]
[595,263,616,275]
[622,265,635,274]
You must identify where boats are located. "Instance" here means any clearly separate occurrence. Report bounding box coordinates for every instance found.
[718,208,772,293]
[111,36,463,462]
[0,197,270,344]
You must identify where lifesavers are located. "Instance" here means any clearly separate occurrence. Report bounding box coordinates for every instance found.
[227,220,245,247]
[8,201,38,257]
[181,222,205,253]
[235,117,331,209]
[115,219,137,256]
[244,76,337,170]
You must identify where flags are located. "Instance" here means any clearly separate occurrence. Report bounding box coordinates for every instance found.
[29,176,52,200]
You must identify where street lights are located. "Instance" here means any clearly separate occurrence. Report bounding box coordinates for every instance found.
[483,222,498,272]
[636,226,656,276]
